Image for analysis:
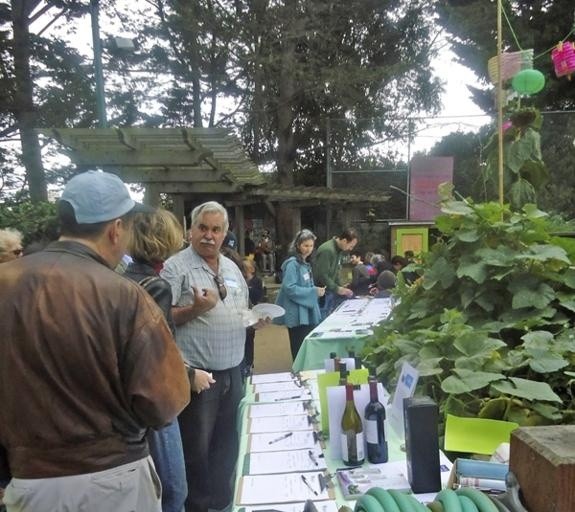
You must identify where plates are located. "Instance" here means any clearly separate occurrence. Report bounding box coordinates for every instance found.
[252,304,286,319]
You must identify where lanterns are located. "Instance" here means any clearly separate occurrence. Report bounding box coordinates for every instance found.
[512,69,545,96]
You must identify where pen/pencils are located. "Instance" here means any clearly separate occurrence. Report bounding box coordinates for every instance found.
[309,451,318,466]
[275,396,300,401]
[302,476,318,495]
[269,433,292,444]
[353,322,374,325]
[343,310,356,312]
[324,286,326,290]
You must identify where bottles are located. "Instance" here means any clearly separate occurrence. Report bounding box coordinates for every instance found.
[329,351,388,466]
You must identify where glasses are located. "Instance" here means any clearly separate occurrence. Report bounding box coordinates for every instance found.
[213,274,227,301]
[12,249,23,256]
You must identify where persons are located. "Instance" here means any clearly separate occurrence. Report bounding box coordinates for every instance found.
[116,207,216,512]
[220,231,263,395]
[258,228,273,271]
[271,225,421,360]
[367,208,376,225]
[0,228,23,261]
[161,201,249,512]
[1,170,190,512]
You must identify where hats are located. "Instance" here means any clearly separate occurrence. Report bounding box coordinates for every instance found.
[222,230,238,252]
[59,169,156,225]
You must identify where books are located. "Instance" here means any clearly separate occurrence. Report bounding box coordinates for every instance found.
[336,448,509,503]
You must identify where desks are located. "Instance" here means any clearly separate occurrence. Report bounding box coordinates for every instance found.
[224,369,454,512]
[291,292,394,374]
